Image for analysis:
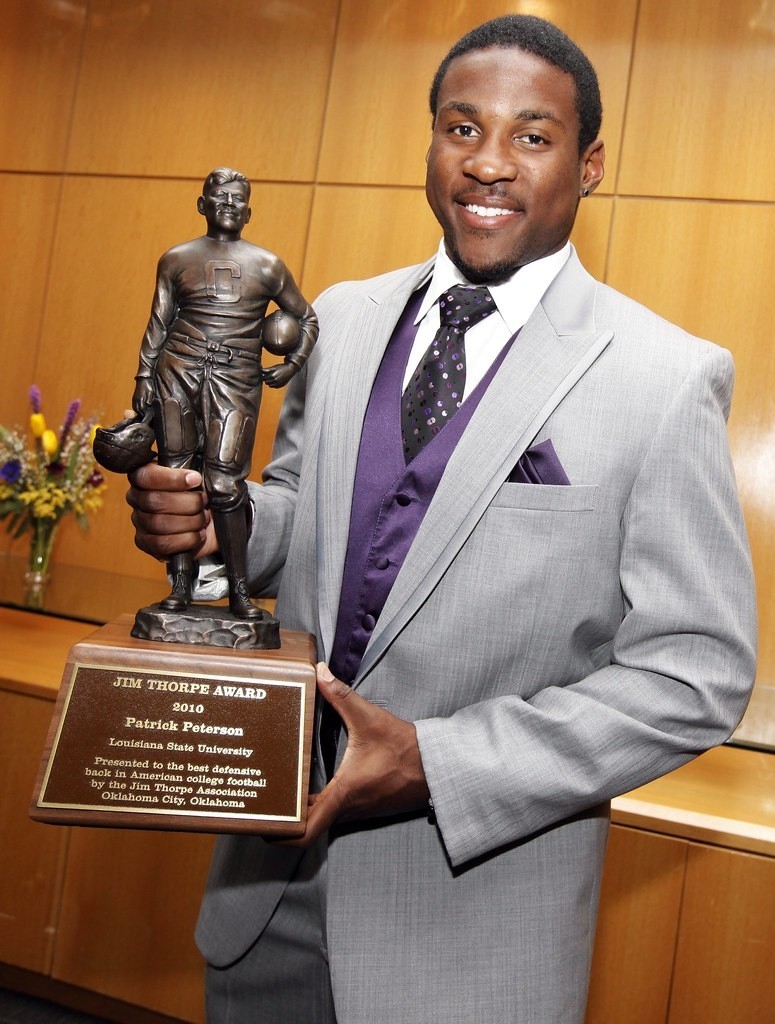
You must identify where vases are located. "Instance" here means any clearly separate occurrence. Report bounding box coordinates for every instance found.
[23,535,58,611]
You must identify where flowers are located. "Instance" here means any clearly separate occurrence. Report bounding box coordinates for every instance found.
[1,386,106,540]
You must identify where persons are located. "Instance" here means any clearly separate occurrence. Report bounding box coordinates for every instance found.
[126,14,756,1024]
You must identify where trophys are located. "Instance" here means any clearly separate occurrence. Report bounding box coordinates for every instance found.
[25,169,321,835]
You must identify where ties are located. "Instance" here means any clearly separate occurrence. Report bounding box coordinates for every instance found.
[401,289,499,466]
